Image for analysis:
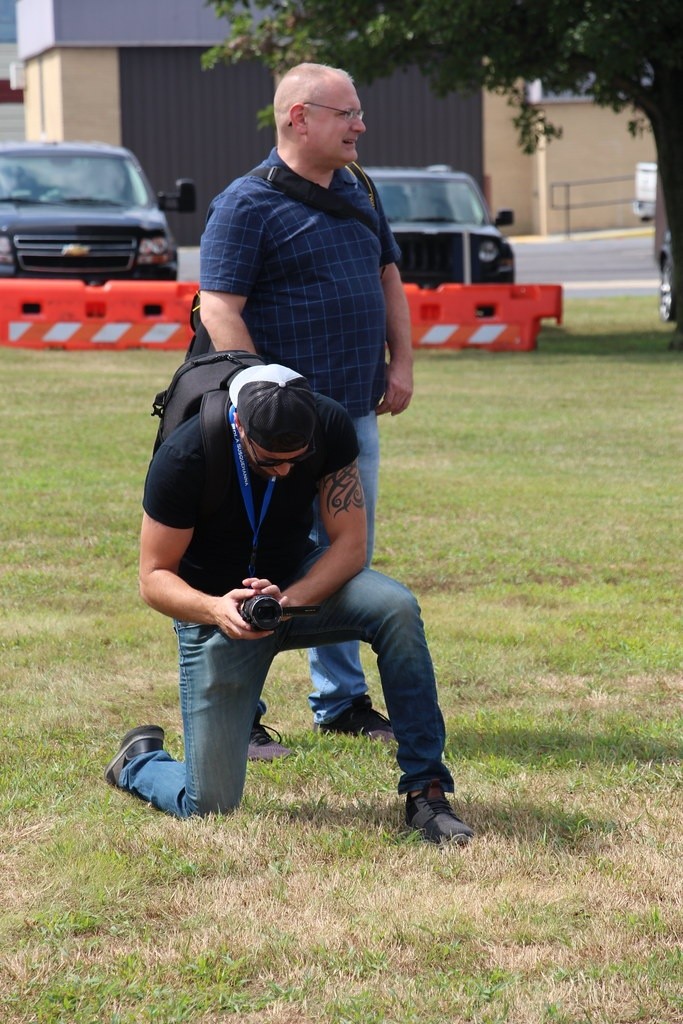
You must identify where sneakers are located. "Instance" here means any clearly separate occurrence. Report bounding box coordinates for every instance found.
[104,725,165,790]
[313,695,397,742]
[247,700,291,759]
[403,779,476,847]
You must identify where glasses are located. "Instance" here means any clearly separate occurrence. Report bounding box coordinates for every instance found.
[289,102,365,123]
[245,433,315,467]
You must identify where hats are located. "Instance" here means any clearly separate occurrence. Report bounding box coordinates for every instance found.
[228,363,316,452]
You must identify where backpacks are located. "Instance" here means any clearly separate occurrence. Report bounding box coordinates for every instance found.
[149,349,266,524]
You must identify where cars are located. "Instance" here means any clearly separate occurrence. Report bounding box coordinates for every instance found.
[361,162,516,284]
[2,140,198,281]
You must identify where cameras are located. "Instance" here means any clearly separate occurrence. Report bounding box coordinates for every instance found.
[238,594,321,631]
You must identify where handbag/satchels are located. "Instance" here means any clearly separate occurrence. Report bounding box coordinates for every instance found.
[184,289,218,361]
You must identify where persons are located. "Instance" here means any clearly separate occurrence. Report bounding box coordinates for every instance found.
[200,62,413,760]
[105,364,473,847]
